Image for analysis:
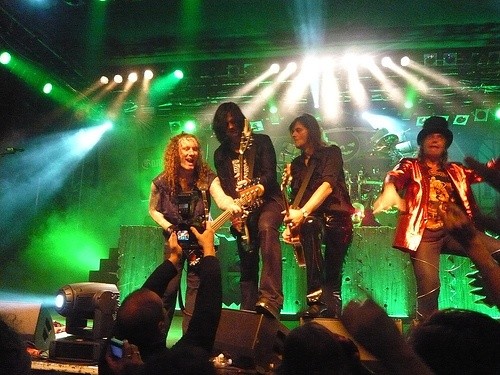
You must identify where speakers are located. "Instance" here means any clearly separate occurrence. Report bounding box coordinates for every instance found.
[299,317,403,362]
[211,308,291,372]
[0,301,55,352]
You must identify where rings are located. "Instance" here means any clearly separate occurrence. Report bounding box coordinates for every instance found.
[133,351,137,354]
[137,351,139,354]
[126,355,132,358]
[290,223,294,226]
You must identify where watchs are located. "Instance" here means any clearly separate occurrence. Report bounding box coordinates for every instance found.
[302,207,309,220]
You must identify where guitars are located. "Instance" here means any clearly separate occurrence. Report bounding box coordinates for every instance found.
[233,119,255,252]
[186,177,264,269]
[280,164,306,269]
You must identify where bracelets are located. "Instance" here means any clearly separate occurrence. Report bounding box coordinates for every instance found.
[165,224,176,235]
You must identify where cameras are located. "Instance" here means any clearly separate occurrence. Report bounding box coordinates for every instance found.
[172,191,204,246]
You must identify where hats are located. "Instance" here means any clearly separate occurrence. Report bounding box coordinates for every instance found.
[417,116,453,148]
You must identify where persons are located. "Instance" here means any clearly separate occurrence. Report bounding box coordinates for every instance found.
[282,113,354,319]
[0,220,500,375]
[212,102,286,321]
[373,116,500,323]
[150,134,241,336]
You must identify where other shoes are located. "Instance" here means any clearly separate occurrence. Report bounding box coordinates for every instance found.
[315,306,342,319]
[256,298,280,321]
[299,303,321,318]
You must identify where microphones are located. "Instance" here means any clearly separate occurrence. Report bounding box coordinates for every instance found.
[5,148,25,151]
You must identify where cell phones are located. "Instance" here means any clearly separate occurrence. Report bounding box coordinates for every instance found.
[110,338,124,361]
